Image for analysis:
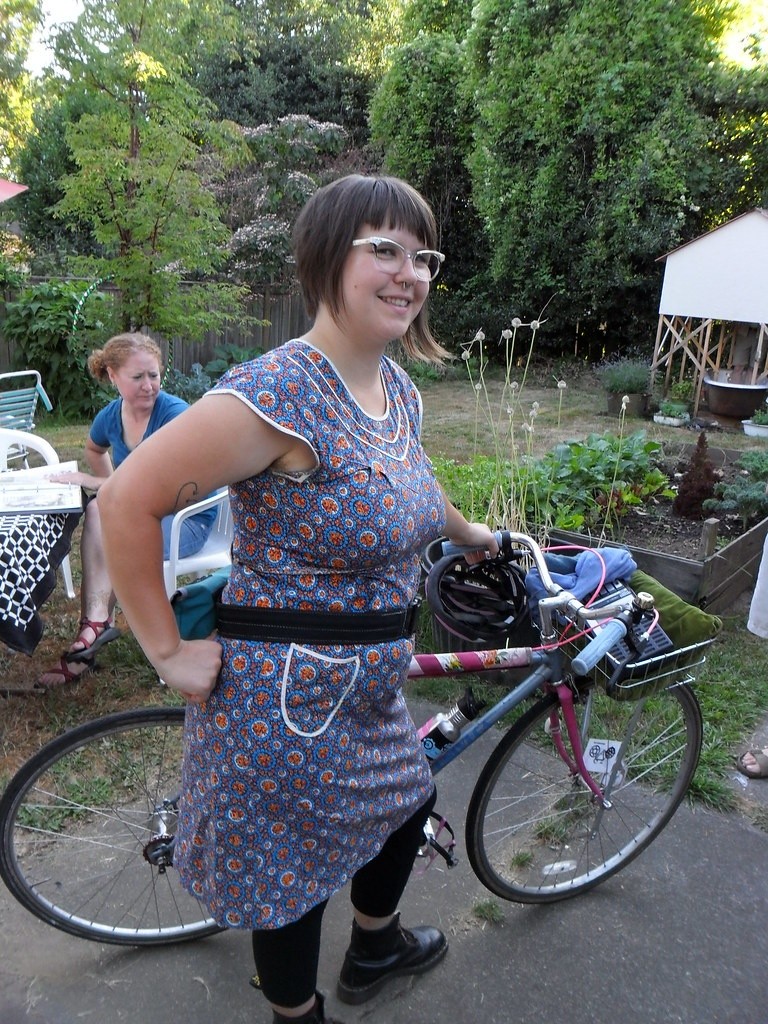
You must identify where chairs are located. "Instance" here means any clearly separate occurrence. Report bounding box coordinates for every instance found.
[0,428,76,599]
[107,485,233,685]
[0,371,53,469]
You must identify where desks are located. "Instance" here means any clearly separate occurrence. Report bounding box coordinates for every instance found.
[0,485,88,696]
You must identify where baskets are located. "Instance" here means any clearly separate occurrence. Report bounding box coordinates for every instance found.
[529,601,719,701]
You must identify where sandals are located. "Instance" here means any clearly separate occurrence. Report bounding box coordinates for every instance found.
[66,616,119,664]
[33,654,94,690]
[737,749,768,780]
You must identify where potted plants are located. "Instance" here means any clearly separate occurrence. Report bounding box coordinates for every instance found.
[601,359,652,416]
[669,381,692,412]
[654,402,687,427]
[741,409,768,437]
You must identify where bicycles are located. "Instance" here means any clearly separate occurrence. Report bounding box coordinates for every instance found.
[1,528,730,950]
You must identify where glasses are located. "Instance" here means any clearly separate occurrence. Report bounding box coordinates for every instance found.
[351,236,446,281]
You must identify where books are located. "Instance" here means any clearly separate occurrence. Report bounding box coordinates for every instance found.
[0,459,83,513]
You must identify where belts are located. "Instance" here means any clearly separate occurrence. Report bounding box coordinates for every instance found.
[213,596,421,645]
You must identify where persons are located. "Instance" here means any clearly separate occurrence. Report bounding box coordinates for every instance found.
[96,173,501,1024]
[734,535,768,781]
[727,321,768,386]
[32,332,221,690]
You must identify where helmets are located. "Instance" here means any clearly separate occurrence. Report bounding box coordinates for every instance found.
[424,551,527,654]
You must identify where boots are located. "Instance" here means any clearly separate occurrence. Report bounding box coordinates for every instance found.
[337,913,449,1005]
[271,992,341,1024]
